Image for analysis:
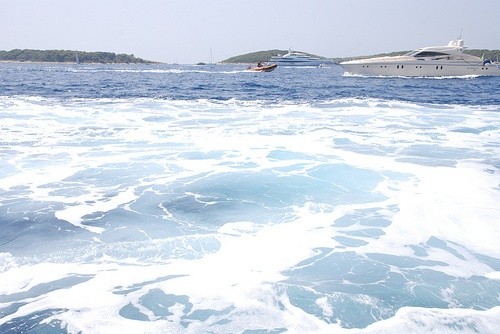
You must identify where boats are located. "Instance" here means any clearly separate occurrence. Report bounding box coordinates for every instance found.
[262,51,325,67]
[336,38,499,79]
[247,63,277,73]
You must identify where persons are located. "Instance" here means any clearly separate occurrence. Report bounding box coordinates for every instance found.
[258,61,269,68]
